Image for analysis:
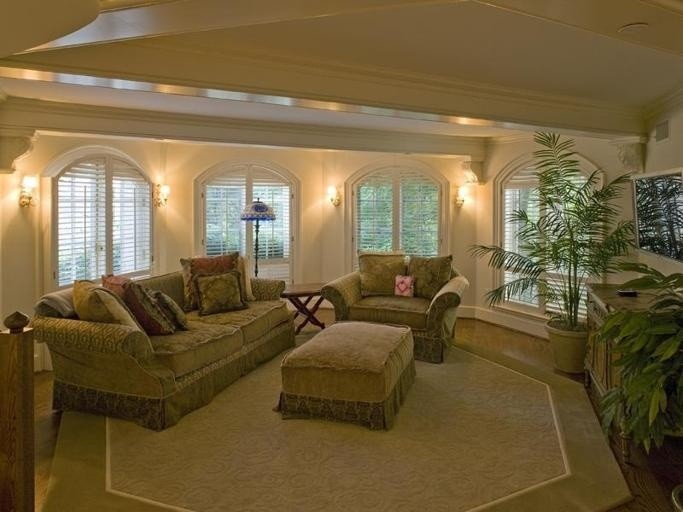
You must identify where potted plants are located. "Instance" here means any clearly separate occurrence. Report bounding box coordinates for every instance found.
[599,261,683,512]
[464,131,635,376]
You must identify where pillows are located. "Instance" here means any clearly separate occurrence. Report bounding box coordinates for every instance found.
[358,254,454,298]
[73,250,256,330]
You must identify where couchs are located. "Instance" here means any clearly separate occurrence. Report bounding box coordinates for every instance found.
[26,267,296,430]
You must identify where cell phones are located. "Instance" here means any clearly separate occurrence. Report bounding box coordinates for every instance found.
[617,288,638,297]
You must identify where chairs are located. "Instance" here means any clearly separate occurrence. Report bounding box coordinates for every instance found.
[320,266,470,365]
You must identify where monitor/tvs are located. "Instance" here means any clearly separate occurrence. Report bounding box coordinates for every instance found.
[630,167,683,267]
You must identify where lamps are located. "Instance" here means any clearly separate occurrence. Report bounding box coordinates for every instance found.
[240,198,276,278]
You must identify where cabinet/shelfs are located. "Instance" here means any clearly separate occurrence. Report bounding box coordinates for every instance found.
[583,282,670,463]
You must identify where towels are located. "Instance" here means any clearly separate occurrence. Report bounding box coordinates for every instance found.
[32,283,77,316]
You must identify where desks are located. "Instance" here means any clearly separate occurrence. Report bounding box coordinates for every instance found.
[280,282,329,335]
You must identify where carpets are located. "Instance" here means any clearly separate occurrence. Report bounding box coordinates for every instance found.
[41,323,632,512]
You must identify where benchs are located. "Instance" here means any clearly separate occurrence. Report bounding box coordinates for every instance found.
[273,319,417,430]
